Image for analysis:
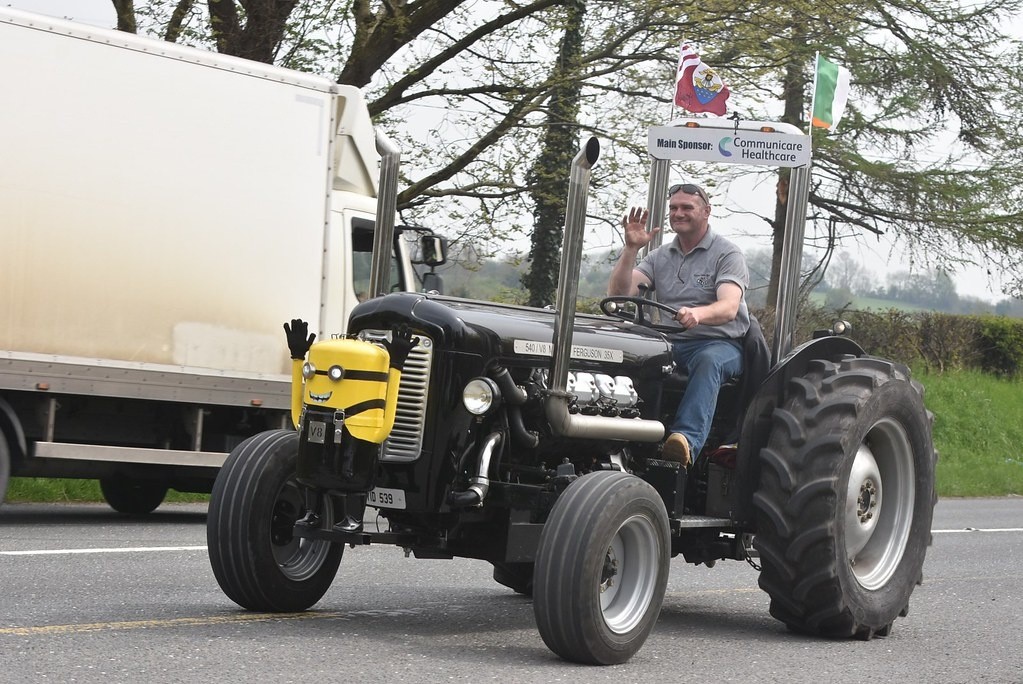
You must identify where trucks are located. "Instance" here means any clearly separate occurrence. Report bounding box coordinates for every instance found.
[1,5,455,515]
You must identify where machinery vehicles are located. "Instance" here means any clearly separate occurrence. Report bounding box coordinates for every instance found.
[205,116,938,670]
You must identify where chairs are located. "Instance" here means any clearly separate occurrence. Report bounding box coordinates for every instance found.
[638,314,771,412]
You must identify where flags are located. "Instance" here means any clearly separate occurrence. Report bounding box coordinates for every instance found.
[812,54,849,132]
[672,41,730,116]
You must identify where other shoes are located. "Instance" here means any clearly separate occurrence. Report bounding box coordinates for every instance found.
[660,432,691,469]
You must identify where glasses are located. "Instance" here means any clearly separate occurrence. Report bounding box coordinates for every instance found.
[668,184,708,206]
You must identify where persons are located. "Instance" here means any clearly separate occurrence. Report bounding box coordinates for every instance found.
[606,183,750,469]
